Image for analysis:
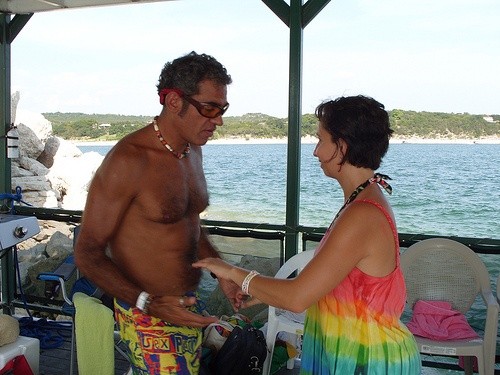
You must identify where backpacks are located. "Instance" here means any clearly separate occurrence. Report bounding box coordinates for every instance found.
[198,325,270,375]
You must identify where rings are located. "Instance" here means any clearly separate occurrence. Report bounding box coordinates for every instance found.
[180,295,187,307]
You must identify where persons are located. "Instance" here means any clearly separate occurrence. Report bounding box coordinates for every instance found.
[74,50,249,375]
[191,94,422,375]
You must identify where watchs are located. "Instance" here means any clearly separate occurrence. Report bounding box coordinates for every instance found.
[135,292,149,311]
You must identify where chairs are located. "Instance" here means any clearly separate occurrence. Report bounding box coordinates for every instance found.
[262,249,317,374]
[37,226,131,375]
[398,236,498,375]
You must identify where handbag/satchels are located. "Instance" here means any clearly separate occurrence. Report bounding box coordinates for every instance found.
[202,309,256,353]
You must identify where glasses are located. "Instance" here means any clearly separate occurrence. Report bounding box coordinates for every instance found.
[159,88,230,119]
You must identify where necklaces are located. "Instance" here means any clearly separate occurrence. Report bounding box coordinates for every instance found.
[154,116,192,159]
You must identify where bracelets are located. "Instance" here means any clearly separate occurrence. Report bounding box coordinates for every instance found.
[241,270,260,295]
[143,295,154,314]
